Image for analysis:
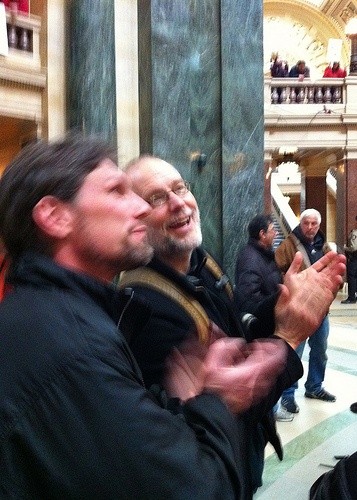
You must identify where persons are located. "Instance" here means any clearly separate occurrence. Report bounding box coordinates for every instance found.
[234,214,294,422]
[269,51,288,104]
[323,61,346,94]
[1,0,29,49]
[289,60,310,94]
[0,135,286,500]
[274,209,337,412]
[341,215,357,304]
[114,154,346,500]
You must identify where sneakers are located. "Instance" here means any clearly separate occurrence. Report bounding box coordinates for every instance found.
[282,397,300,413]
[273,406,295,422]
[305,388,337,402]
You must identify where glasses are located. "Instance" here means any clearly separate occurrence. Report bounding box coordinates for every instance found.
[147,179,191,206]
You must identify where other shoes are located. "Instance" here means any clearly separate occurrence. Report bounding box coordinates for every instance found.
[341,298,356,303]
